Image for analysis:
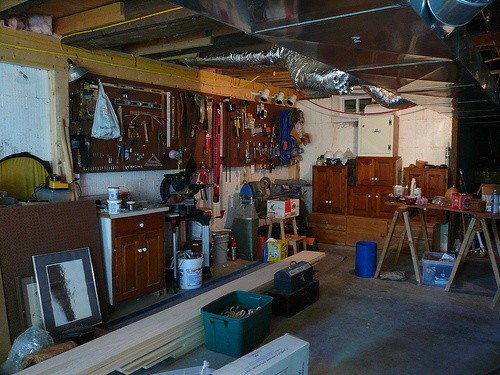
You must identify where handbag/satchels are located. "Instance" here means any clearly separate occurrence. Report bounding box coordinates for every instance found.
[90,82,120,140]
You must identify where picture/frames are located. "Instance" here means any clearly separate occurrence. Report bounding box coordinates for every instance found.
[32,247,103,337]
[15,271,44,334]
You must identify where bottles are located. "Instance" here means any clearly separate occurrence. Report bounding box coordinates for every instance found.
[491,190,499,213]
[230,238,237,261]
[411,178,416,196]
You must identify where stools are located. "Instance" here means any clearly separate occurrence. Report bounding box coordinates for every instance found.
[268,217,298,240]
[286,236,307,255]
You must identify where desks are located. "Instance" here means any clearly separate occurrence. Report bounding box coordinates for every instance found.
[374,201,500,292]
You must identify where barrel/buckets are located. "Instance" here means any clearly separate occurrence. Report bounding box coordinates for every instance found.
[106,187,122,215]
[177,254,205,290]
[214,234,230,264]
[355,241,377,278]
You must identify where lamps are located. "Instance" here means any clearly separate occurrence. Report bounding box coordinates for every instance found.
[250,89,298,106]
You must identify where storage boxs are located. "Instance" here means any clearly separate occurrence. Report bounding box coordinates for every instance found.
[267,199,299,219]
[422,251,456,287]
[36,188,72,203]
[453,192,472,210]
[267,240,288,263]
[200,291,273,358]
[477,184,500,206]
[211,333,310,375]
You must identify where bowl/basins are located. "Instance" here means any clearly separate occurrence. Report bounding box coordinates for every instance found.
[404,196,417,204]
[432,196,445,205]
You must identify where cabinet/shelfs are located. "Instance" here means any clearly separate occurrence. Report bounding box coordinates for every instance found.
[309,114,449,255]
[98,203,169,306]
